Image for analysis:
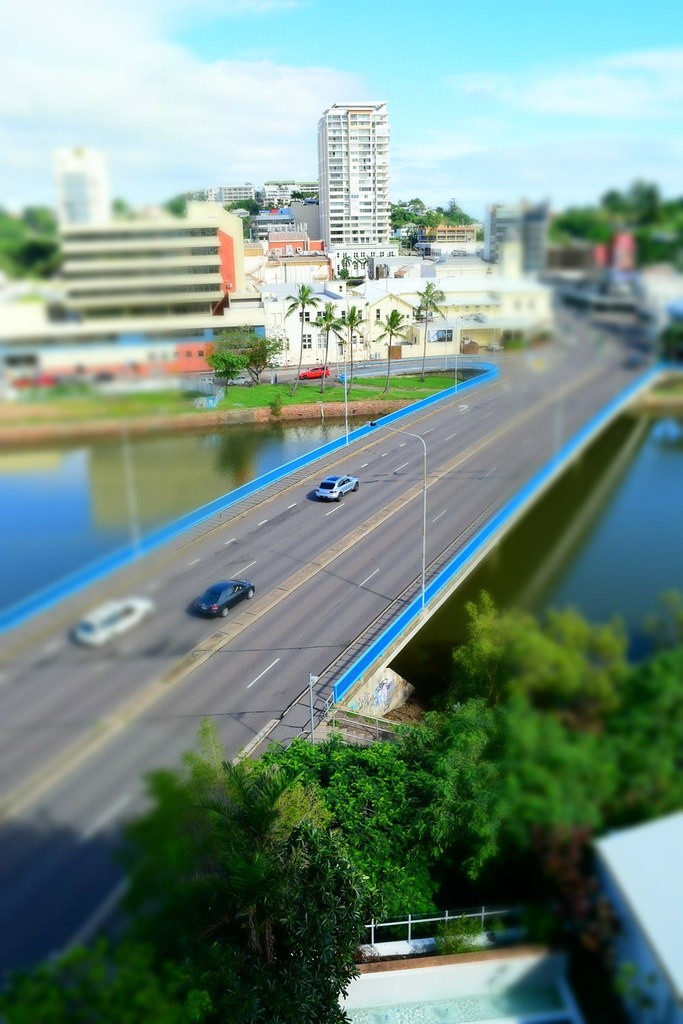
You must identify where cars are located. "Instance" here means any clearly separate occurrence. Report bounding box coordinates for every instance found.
[187,578,257,619]
[298,364,331,380]
[226,376,253,387]
[313,474,360,502]
[71,595,156,648]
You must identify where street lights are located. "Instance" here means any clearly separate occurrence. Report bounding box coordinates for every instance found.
[370,422,427,608]
